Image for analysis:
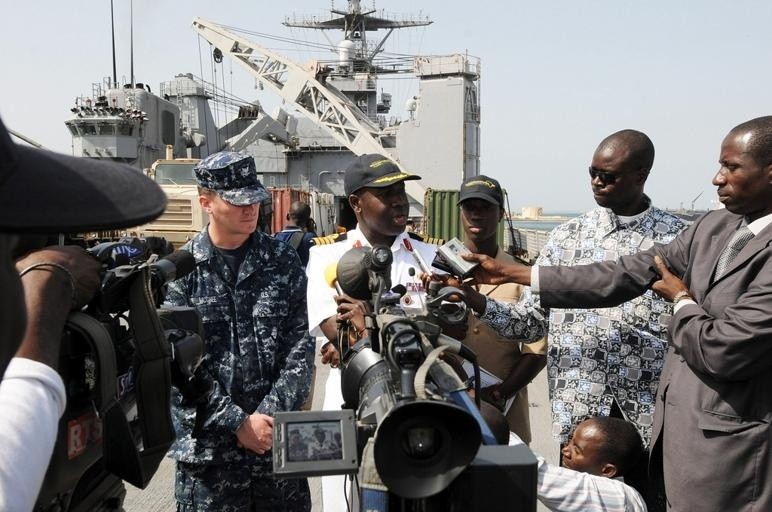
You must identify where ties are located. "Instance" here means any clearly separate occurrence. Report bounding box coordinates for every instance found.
[711,226,755,285]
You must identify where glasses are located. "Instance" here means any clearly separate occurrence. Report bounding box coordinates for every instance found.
[589,167,644,183]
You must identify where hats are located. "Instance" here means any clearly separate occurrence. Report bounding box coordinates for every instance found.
[456,175,502,208]
[290,430,298,436]
[193,151,270,206]
[344,155,422,195]
[314,427,326,435]
[0,115,169,231]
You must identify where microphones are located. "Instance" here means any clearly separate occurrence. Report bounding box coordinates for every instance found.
[431,252,481,280]
[436,238,481,278]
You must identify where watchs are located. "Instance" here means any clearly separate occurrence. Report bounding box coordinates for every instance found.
[673,292,689,304]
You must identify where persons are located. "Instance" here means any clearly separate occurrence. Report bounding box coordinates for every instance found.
[161,150,314,512]
[0,246,101,511]
[308,428,333,459]
[305,152,449,512]
[417,130,693,466]
[506,417,648,512]
[331,432,340,447]
[461,115,772,512]
[289,428,308,455]
[449,176,548,447]
[271,199,313,262]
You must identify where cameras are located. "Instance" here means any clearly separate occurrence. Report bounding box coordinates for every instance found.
[32,230,211,512]
[275,244,538,512]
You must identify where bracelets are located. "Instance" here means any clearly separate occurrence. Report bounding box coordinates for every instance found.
[18,261,78,297]
